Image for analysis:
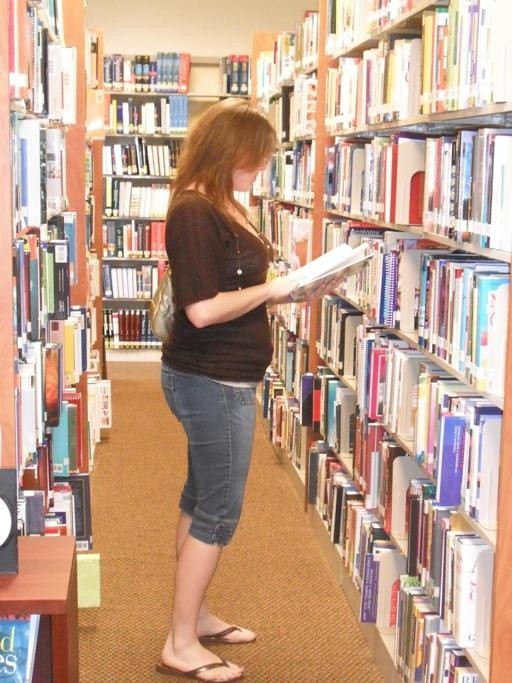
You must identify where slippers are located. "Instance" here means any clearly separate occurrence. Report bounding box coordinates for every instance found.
[155,651,246,683]
[195,624,257,644]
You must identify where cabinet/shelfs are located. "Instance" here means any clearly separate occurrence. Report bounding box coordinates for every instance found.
[2,0,510,681]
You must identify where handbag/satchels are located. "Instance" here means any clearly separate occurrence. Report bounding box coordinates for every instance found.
[149,260,178,341]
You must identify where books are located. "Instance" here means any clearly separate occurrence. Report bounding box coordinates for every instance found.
[104,52,194,350]
[0,0,113,683]
[251,1,512,683]
[219,55,250,102]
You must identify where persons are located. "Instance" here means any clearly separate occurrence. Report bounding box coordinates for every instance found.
[149,93,345,682]
